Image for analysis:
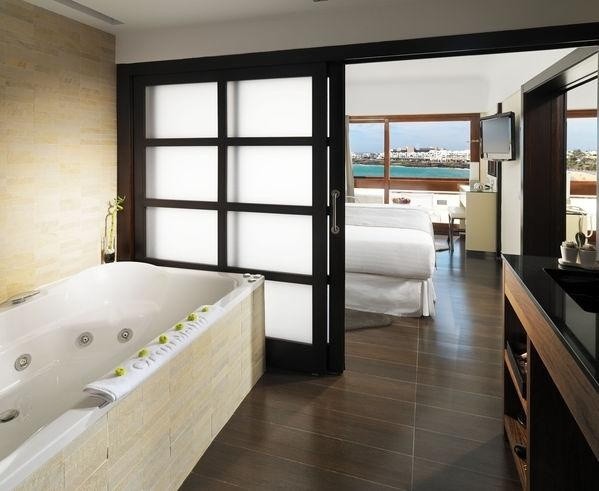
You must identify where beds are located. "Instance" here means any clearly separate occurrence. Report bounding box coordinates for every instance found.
[344,203,436,320]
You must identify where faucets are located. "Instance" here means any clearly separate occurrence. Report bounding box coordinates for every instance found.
[9,289,41,304]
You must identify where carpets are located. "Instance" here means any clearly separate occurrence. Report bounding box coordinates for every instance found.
[433,241,450,253]
[344,307,392,332]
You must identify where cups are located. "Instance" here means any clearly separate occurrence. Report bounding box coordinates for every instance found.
[560,246,579,263]
[579,249,599,266]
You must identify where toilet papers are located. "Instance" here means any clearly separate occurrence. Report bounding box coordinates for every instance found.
[469,161,480,181]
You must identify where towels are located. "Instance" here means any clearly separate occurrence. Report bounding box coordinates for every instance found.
[83,305,228,408]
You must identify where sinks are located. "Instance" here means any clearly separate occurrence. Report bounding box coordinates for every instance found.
[542,266,598,315]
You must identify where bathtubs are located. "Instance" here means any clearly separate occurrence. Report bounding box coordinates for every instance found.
[0,259,267,491]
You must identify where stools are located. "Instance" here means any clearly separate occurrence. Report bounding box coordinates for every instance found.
[446,206,465,252]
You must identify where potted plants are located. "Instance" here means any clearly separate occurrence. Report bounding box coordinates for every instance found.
[560,240,598,265]
[101,193,127,263]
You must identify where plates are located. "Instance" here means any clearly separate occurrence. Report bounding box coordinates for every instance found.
[557,259,580,267]
[580,264,599,271]
[558,263,579,271]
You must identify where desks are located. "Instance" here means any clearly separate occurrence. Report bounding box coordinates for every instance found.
[459,185,499,253]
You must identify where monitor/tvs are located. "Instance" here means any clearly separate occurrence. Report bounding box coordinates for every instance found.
[480,111,516,162]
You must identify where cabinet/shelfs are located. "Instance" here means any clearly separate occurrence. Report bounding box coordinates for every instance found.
[497,261,599,489]
